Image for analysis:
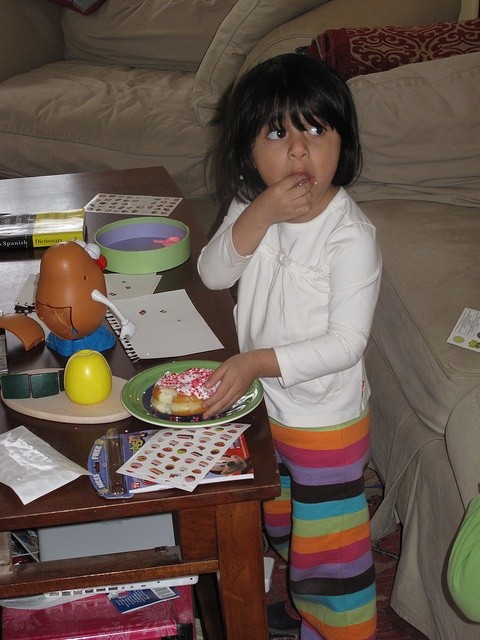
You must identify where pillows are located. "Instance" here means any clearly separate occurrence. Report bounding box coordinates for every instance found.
[188,0,321,125]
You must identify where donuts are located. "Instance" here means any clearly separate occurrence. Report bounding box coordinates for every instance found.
[150,368,225,416]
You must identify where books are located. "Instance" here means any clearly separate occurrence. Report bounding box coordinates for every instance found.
[0,207,86,250]
[119,427,255,495]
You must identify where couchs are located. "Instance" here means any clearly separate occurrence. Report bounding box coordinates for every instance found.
[0,0,461,187]
[339,51,480,640]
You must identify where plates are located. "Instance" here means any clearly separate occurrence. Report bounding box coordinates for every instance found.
[121,359,264,428]
[1,367,133,426]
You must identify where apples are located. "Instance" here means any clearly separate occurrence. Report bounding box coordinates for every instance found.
[64,350,112,405]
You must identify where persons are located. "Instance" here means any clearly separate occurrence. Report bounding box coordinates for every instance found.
[195,54,384,640]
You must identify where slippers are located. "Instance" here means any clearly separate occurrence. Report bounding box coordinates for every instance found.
[267,601,301,635]
